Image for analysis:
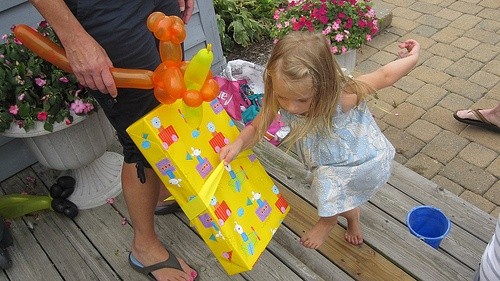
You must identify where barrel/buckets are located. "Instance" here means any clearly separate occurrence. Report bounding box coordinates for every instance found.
[407,206,451,249]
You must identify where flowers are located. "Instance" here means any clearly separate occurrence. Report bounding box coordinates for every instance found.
[270,0,386,56]
[0,20,99,132]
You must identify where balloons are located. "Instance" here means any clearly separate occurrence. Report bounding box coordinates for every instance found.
[0,173,78,225]
[11,11,219,109]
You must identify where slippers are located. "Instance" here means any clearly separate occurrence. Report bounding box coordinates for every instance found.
[453,108,500,134]
[154,201,183,215]
[129,248,199,281]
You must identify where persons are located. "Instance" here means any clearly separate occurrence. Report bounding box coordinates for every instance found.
[219,32,420,249]
[20,0,199,281]
[452,99,500,131]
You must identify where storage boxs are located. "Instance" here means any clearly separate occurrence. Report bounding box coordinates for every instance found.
[126,99,291,275]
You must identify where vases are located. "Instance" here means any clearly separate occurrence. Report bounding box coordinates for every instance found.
[0,108,125,211]
[332,49,357,73]
